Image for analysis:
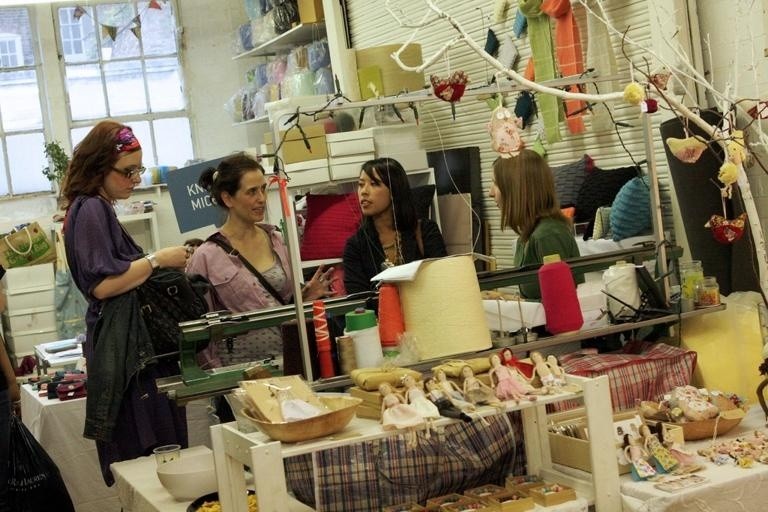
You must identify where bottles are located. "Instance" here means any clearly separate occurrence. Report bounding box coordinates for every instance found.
[681,261,720,306]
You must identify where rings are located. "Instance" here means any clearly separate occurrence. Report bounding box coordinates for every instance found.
[320,277,326,283]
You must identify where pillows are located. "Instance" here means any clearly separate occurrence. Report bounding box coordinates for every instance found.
[550,153,651,241]
[412,184,436,219]
[300,192,362,261]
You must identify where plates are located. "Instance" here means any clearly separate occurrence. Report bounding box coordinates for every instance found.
[670,410,745,440]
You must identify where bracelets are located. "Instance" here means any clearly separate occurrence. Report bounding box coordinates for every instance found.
[144,252,160,274]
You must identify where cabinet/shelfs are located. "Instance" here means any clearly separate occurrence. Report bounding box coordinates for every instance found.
[288,169,444,286]
[54,211,160,252]
[110,243,768,512]
[230,23,326,126]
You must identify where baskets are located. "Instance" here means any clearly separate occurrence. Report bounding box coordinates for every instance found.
[638,385,750,440]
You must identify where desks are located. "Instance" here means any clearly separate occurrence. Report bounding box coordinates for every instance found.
[32,339,86,374]
[21,384,220,512]
[575,232,671,280]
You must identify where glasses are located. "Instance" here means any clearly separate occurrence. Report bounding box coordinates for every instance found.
[110,166,147,180]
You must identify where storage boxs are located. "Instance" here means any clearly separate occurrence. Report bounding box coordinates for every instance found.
[356,43,426,99]
[260,93,428,189]
[1,263,57,368]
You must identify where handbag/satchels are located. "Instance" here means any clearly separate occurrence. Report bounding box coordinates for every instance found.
[137,272,213,361]
[28,369,88,401]
[0,412,76,512]
[0,221,57,271]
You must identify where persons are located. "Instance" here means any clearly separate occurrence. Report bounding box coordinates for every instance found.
[343,156,448,324]
[63,118,193,489]
[619,386,768,479]
[186,149,341,425]
[375,345,565,449]
[0,336,22,466]
[489,148,585,300]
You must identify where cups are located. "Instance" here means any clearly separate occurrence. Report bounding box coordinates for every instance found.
[150,445,180,464]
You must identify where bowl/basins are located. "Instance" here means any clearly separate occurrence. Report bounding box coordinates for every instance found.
[156,456,224,501]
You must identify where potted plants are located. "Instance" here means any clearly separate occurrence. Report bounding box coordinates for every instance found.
[42,142,71,222]
